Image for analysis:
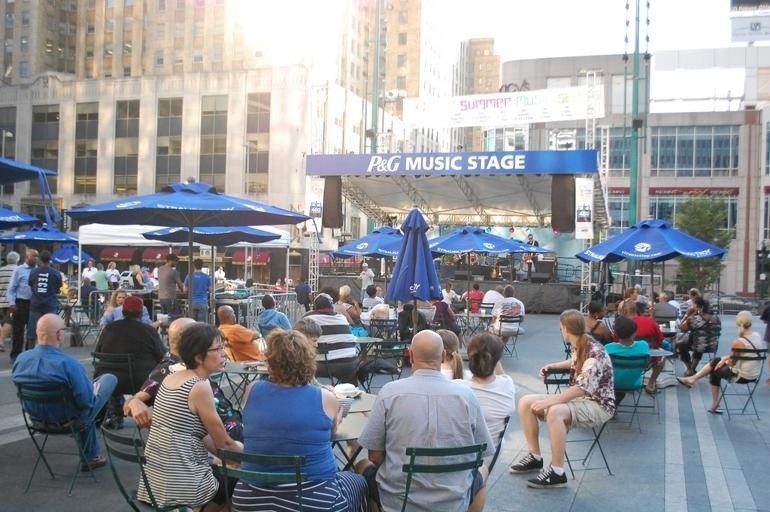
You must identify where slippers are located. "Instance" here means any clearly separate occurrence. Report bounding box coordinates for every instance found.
[676,376,693,389]
[647,388,661,394]
[708,408,723,414]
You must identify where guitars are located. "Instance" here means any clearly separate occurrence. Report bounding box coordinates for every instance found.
[453,253,467,261]
[523,253,539,261]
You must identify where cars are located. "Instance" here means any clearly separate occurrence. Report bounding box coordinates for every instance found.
[704,289,727,299]
[712,295,761,315]
[119,270,134,285]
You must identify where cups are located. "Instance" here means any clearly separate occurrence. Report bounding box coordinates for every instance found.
[464,308,467,315]
[480,309,486,316]
[670,320,675,330]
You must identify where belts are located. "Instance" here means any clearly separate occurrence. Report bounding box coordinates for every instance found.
[29,416,76,426]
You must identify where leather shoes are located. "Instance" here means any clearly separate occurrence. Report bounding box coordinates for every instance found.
[81,455,107,472]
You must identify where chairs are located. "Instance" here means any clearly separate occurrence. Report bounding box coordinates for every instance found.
[559,300,720,434]
[713,348,769,420]
[14,299,526,512]
[541,369,614,481]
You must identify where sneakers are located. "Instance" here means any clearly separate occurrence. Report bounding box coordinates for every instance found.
[526,465,568,489]
[510,453,544,473]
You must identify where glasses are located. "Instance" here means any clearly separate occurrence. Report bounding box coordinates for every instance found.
[209,347,224,352]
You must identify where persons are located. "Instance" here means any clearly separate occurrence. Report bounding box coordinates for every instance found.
[395,280,525,336]
[217,264,391,386]
[227,332,369,512]
[676,310,763,414]
[507,310,616,489]
[363,329,514,511]
[2,249,63,360]
[82,253,212,324]
[125,318,244,510]
[10,314,120,469]
[586,285,721,420]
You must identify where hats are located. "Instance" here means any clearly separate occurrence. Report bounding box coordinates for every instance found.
[614,315,638,339]
[124,296,143,313]
